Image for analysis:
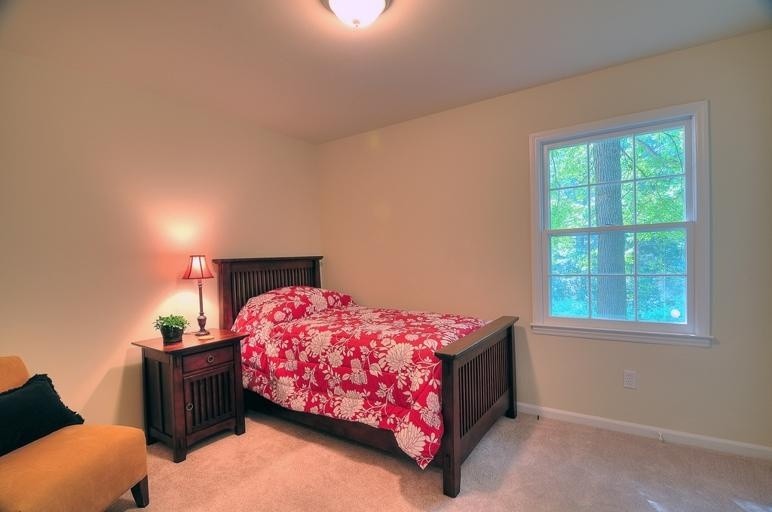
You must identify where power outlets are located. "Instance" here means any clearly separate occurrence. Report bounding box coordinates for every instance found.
[623,371,637,389]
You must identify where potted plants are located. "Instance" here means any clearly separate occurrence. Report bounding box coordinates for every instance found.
[153,314,191,343]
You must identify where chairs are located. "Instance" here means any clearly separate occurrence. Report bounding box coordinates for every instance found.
[0,355,150,512]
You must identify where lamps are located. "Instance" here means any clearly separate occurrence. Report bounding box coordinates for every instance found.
[183,255,215,336]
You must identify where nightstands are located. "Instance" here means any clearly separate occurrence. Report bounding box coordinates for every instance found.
[129,328,250,463]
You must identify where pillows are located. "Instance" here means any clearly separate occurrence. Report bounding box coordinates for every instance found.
[1,373,84,457]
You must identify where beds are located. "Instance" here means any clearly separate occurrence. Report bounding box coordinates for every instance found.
[213,256,519,499]
